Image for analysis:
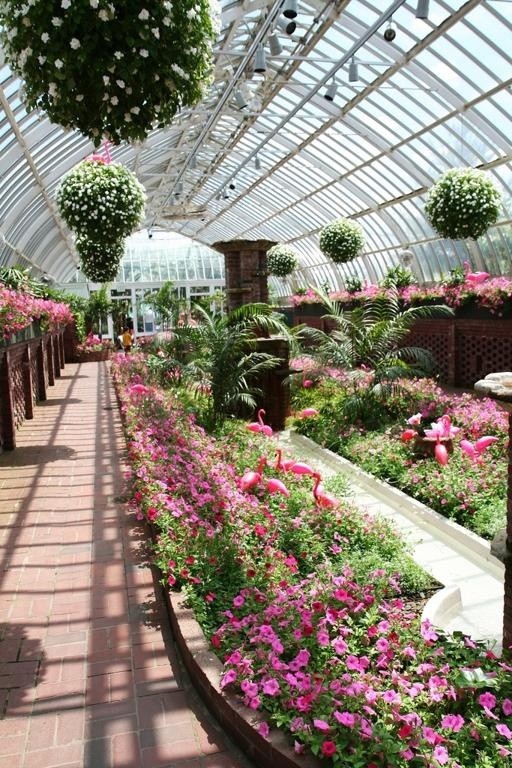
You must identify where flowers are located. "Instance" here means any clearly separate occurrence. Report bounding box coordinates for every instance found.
[411,377,451,436]
[74,237,124,283]
[2,2,217,141]
[56,160,147,237]
[262,168,512,319]
[1,288,74,339]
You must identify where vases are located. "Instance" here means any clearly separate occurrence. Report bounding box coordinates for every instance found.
[413,432,453,461]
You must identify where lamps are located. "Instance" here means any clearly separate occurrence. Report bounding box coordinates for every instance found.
[231,0,429,111]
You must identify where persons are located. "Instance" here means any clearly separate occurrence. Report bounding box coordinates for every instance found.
[115,318,133,351]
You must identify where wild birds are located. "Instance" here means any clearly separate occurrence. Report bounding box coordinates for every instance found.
[424,414,461,438]
[434,432,450,467]
[459,428,500,463]
[293,409,320,419]
[302,370,313,388]
[246,408,272,437]
[237,448,313,499]
[130,384,148,399]
[463,261,491,284]
[307,472,339,509]
